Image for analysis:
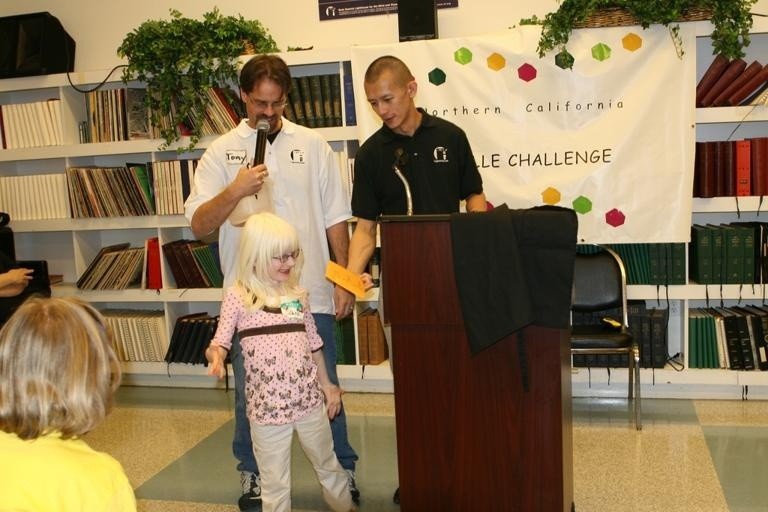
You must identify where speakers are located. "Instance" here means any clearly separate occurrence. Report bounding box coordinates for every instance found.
[398,0,438,42]
[0,11,76,79]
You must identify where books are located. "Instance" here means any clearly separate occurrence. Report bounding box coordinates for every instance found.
[285,74,356,128]
[68,85,242,218]
[698,136,767,198]
[333,247,387,365]
[691,221,768,285]
[697,55,768,106]
[80,236,228,363]
[2,100,68,219]
[688,305,768,372]
[575,243,685,368]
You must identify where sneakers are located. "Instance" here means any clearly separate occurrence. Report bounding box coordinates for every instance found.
[345,469,360,501]
[236,468,263,511]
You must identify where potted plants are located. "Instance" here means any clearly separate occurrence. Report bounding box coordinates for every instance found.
[114,5,322,154]
[508,0,760,72]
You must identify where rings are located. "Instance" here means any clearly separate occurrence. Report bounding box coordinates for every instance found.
[348,305,354,310]
[261,171,265,175]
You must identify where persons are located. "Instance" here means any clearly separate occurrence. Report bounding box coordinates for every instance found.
[344,55,488,292]
[184,56,359,512]
[205,213,358,511]
[1,267,35,298]
[1,297,139,512]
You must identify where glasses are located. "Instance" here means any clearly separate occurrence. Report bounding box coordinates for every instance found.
[273,247,301,261]
[249,94,288,108]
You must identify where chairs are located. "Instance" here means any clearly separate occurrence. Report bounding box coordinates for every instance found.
[566,241,644,431]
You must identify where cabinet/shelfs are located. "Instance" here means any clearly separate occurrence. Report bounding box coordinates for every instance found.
[61,62,191,390]
[688,23,768,402]
[149,48,358,394]
[0,71,105,303]
[358,239,690,399]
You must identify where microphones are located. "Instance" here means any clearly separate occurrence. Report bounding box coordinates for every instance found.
[253,120,270,166]
